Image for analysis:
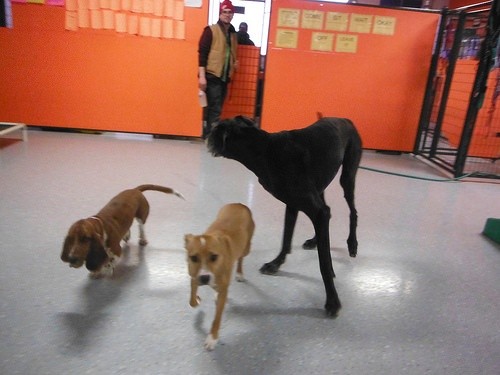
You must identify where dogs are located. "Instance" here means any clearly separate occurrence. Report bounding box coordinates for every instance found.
[206,114,363,319]
[59,183,186,278]
[182,202,256,351]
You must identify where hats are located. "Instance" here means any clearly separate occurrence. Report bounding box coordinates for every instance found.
[220,0,234,13]
[239,22,247,30]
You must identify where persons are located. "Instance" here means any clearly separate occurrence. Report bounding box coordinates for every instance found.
[197,0,239,146]
[233,23,255,45]
